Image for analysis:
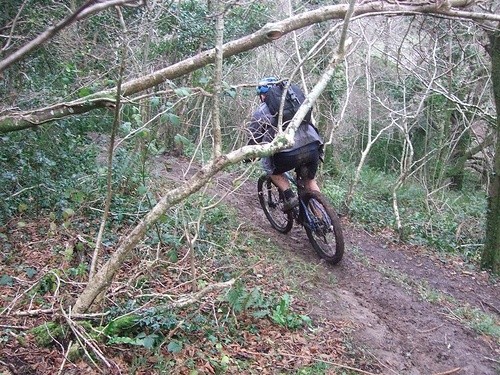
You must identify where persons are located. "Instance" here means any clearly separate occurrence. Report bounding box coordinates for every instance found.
[242,76,334,237]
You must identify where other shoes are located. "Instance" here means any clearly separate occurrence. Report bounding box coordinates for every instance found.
[311,220,328,230]
[282,197,299,213]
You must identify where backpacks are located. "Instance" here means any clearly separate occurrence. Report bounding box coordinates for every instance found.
[263,79,312,128]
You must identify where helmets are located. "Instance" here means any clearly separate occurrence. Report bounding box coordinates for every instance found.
[257,76,279,95]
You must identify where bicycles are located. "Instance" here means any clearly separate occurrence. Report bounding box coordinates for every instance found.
[257,171,345,265]
